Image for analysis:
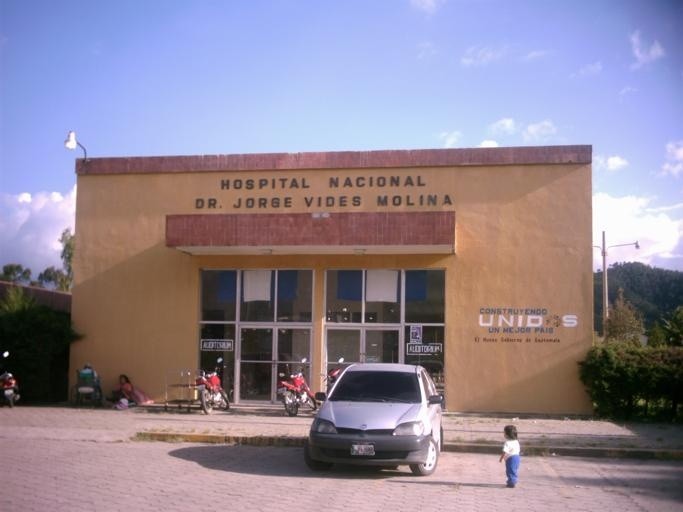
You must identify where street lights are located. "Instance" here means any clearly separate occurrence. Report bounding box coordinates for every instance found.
[602,239,641,337]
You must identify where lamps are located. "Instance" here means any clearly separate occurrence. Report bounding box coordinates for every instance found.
[60,129,91,167]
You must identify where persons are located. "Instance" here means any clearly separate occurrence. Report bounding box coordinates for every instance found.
[113,374,133,402]
[499,424,521,489]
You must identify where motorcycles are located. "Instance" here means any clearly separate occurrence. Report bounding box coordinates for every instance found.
[322,356,349,385]
[274,357,316,418]
[195,356,230,417]
[0,350,21,411]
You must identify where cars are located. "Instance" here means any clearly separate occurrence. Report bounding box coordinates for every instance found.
[301,361,448,480]
[409,359,444,397]
